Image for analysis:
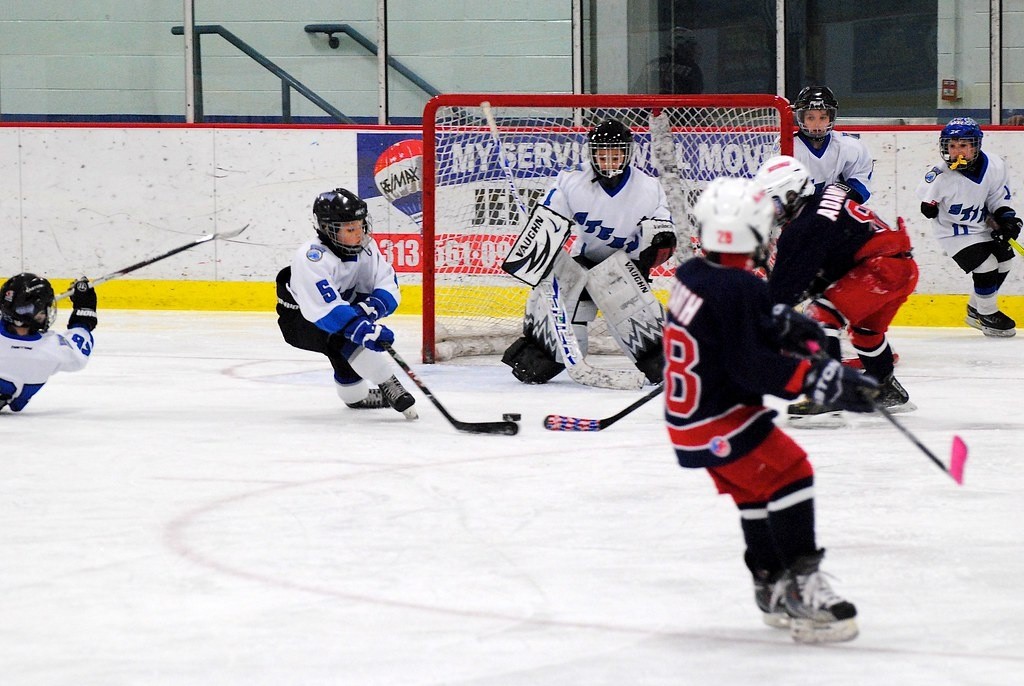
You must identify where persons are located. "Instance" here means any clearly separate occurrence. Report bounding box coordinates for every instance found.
[790,83,870,203]
[276,187,420,421]
[668,177,864,645]
[752,156,918,427]
[499,120,675,394]
[920,116,1024,337]
[0,273,96,412]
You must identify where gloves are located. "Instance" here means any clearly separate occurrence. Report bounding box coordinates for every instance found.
[65,276,98,330]
[992,205,1023,248]
[355,297,388,323]
[344,315,395,353]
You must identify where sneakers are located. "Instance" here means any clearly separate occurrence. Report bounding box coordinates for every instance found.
[378,375,420,420]
[768,547,859,644]
[346,388,391,410]
[976,310,1017,337]
[752,576,791,628]
[965,304,983,331]
[860,371,918,414]
[786,398,843,429]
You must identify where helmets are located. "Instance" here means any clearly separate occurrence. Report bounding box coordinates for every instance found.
[938,117,984,170]
[311,187,374,261]
[0,272,58,334]
[588,120,634,178]
[693,176,774,253]
[755,155,815,227]
[794,84,839,141]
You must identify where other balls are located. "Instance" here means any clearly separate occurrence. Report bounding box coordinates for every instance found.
[503,412,521,422]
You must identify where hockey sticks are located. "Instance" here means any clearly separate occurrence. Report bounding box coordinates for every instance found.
[481,100,646,393]
[55,223,251,301]
[544,384,665,432]
[985,212,1024,258]
[371,320,519,437]
[876,401,968,485]
